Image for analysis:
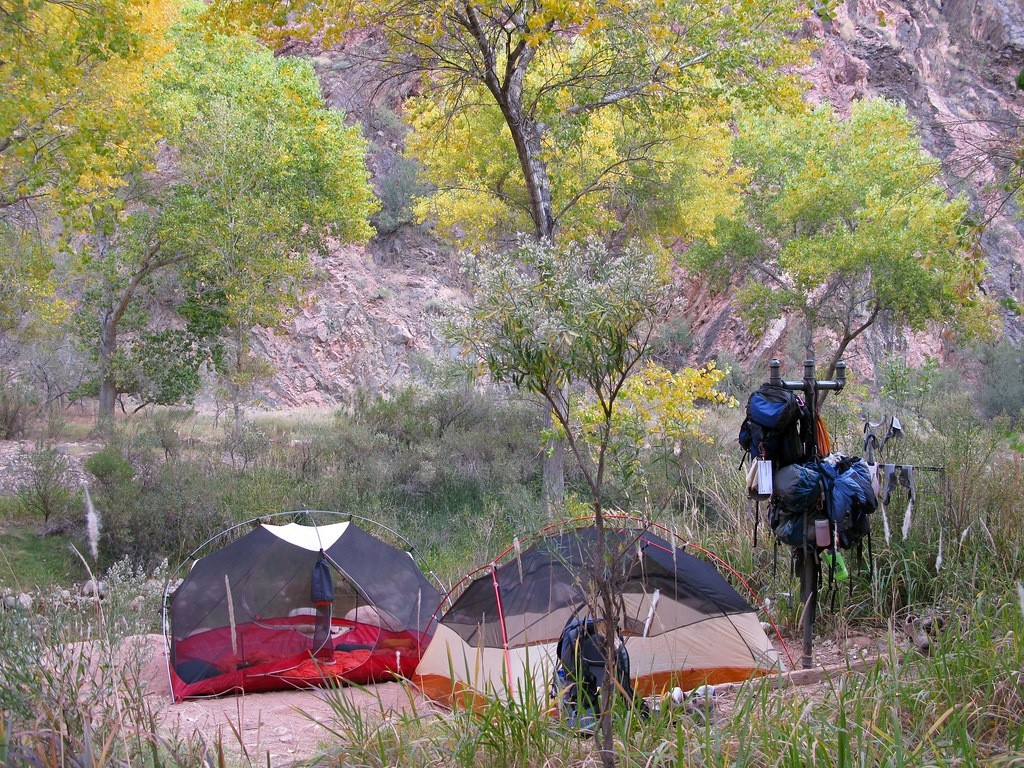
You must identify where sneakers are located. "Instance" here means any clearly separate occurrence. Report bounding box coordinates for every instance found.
[922,616,945,636]
[685,697,715,724]
[903,613,930,648]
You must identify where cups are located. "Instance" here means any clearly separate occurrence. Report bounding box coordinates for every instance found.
[815,519,830,546]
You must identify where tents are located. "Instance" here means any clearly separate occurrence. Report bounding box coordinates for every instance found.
[409,516,794,724]
[165,518,442,707]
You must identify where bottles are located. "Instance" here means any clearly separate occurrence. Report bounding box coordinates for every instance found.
[827,550,848,580]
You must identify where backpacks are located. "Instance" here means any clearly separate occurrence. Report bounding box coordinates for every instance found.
[551,606,650,739]
[738,379,813,472]
